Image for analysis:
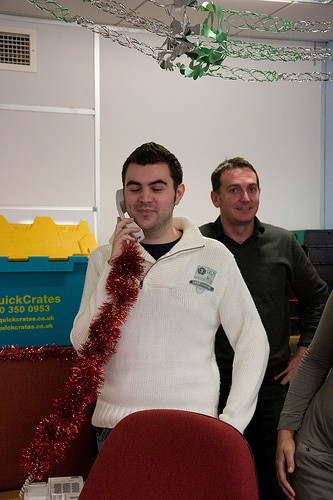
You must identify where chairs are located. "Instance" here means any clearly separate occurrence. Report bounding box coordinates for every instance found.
[76,409,260,500]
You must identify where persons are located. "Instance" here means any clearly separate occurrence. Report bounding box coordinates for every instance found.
[71,142,270,458]
[275,289,333,500]
[197,157,329,500]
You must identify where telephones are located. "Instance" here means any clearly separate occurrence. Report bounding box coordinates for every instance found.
[116,188,145,243]
